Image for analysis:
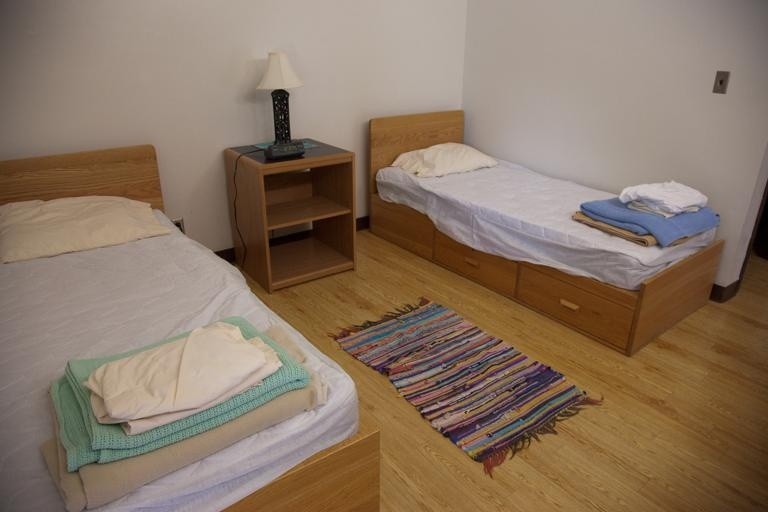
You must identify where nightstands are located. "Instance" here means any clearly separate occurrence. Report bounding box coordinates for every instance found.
[222,139,358,294]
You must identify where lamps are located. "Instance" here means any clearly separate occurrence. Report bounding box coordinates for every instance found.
[254,51,304,144]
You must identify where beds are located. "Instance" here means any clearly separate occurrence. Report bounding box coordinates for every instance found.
[368,110,725,357]
[1,146,381,511]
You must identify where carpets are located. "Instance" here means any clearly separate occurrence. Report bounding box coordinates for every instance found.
[330,296,603,475]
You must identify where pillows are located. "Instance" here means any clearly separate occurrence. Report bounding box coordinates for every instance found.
[0,195,172,264]
[391,141,497,178]
